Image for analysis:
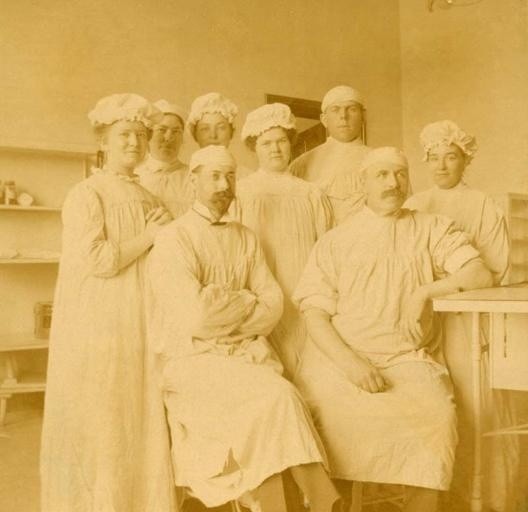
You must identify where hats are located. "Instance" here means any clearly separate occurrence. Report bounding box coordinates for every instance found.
[152,98,186,132]
[186,92,239,135]
[419,119,479,165]
[241,102,297,143]
[320,85,367,114]
[188,144,237,178]
[88,93,164,130]
[356,145,409,180]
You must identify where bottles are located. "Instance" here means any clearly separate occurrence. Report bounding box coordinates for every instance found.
[1,180,17,206]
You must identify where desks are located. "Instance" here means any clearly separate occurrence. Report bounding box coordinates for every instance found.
[434,280,528,509]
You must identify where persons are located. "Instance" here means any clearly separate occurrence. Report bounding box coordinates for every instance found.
[292,146,494,511]
[404,121,515,512]
[237,101,332,378]
[132,100,192,222]
[187,93,245,217]
[38,92,183,512]
[291,86,375,221]
[148,146,342,511]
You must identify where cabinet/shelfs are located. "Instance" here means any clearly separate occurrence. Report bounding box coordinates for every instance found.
[503,184,528,281]
[1,139,108,426]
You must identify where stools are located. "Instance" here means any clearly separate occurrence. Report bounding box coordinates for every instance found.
[177,467,409,511]
